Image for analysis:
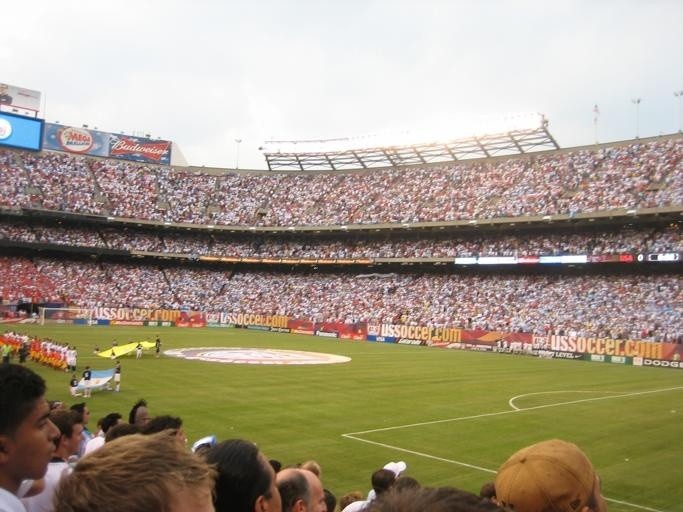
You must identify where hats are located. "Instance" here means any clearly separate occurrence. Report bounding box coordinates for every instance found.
[383,460,406,479]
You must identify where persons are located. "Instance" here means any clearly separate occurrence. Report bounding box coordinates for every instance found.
[1,362,336,512]
[1,330,161,399]
[2,137,681,358]
[339,460,507,510]
